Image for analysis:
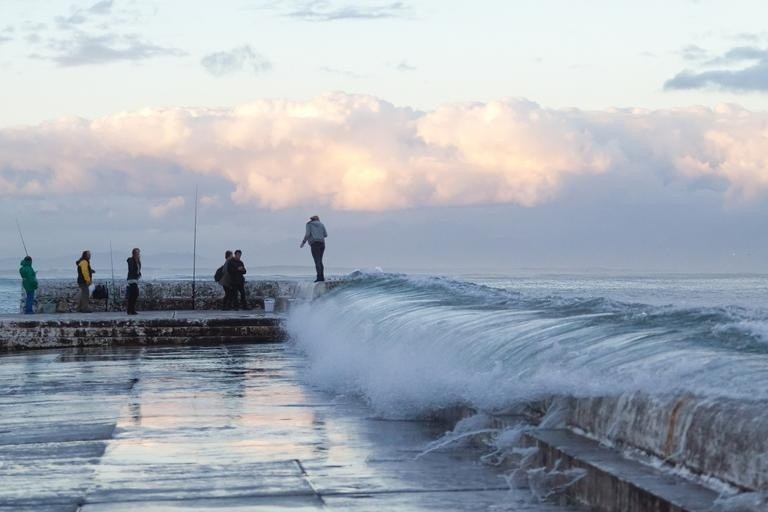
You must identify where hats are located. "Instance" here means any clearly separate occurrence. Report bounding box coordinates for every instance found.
[226,251,232,258]
[24,256,32,262]
[310,216,319,220]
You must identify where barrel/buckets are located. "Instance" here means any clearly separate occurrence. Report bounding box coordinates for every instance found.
[264,298,275,312]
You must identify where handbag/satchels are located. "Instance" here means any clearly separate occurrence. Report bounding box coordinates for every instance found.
[215,266,225,281]
[93,284,107,298]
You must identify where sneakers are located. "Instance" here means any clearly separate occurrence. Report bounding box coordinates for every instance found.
[80,309,92,312]
[223,305,252,310]
[314,279,323,282]
[127,310,137,314]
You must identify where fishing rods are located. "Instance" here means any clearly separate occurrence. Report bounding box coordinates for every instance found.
[16,219,28,256]
[192,185,197,310]
[111,239,116,311]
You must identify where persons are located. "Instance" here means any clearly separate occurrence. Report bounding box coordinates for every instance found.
[19,255,38,314]
[227,249,253,311]
[75,251,96,313]
[218,250,234,311]
[125,248,142,315]
[299,215,327,283]
